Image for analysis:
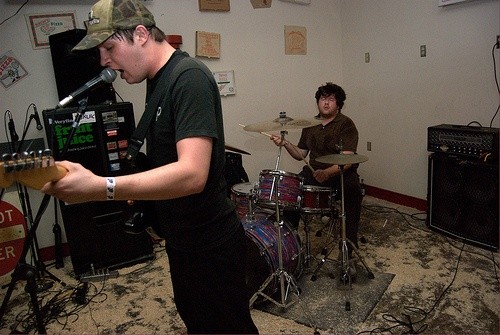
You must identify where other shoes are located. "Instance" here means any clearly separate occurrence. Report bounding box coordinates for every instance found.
[338,242,352,262]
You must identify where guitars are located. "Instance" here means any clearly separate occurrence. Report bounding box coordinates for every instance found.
[0,148,164,240]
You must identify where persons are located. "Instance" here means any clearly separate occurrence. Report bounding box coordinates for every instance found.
[271,83,361,275]
[42,0,260,335]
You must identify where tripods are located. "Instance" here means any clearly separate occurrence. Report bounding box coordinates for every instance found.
[249,123,375,335]
[2,113,67,289]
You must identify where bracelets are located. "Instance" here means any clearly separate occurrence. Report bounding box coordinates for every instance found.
[106,176,115,200]
[284,137,287,146]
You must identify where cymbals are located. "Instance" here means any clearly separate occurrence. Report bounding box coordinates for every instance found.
[242,117,323,132]
[314,154,369,165]
[225,145,251,155]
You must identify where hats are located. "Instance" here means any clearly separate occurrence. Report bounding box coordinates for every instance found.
[71,0,156,51]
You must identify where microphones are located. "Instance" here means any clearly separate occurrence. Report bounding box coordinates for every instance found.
[8,111,19,141]
[55,67,117,110]
[34,105,42,130]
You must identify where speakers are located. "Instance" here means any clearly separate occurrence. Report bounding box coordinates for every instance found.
[426,152,500,252]
[49,28,116,107]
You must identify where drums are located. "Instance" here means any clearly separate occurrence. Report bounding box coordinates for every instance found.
[231,169,334,304]
